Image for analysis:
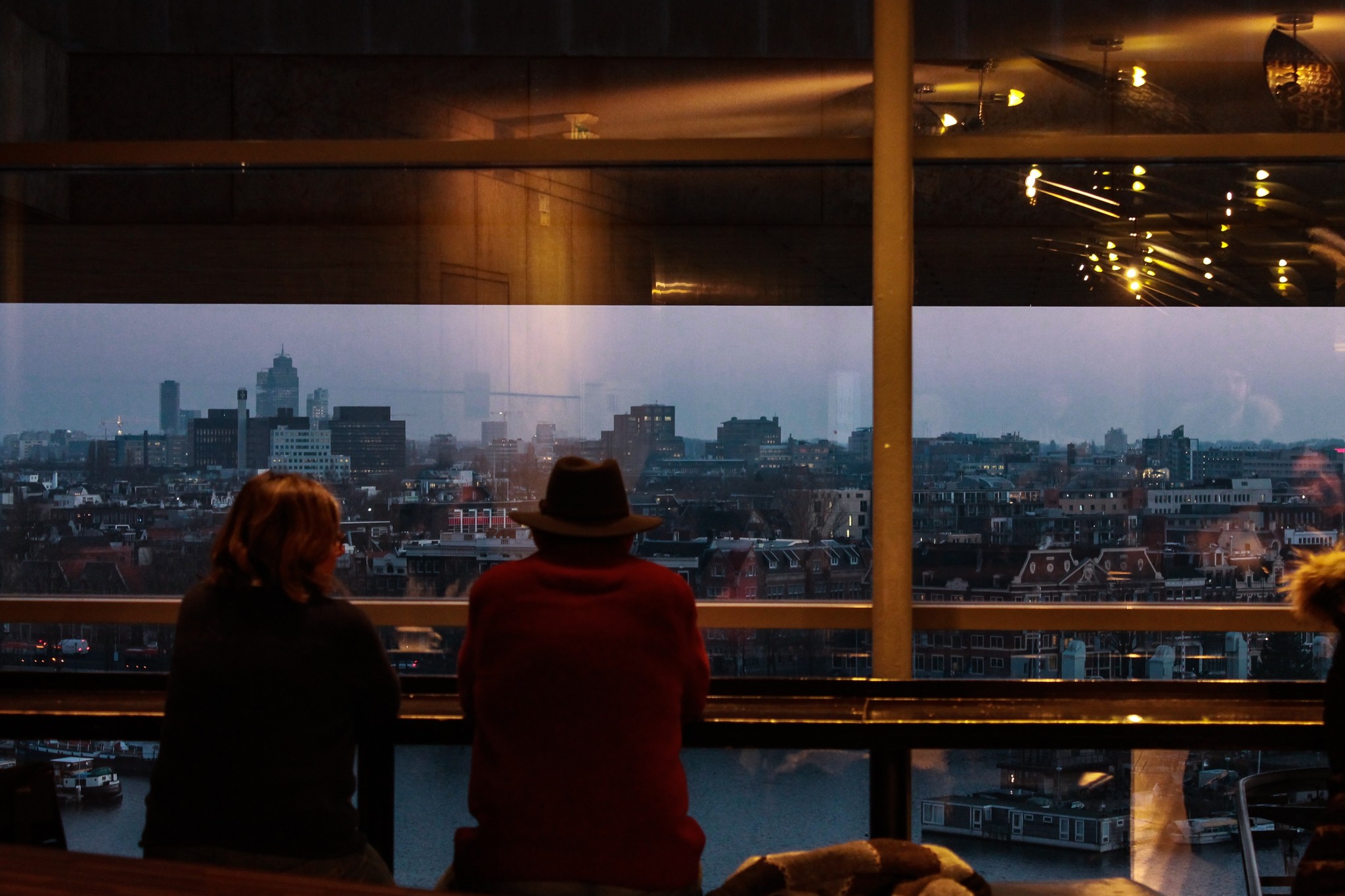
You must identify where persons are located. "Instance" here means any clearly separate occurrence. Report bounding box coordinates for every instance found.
[1275,541,1345,827]
[451,457,715,896]
[1279,451,1345,570]
[137,471,401,885]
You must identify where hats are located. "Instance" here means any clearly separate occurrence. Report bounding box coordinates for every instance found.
[506,456,661,539]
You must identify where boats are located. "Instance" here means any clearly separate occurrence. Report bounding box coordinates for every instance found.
[14,740,161,777]
[45,755,124,807]
